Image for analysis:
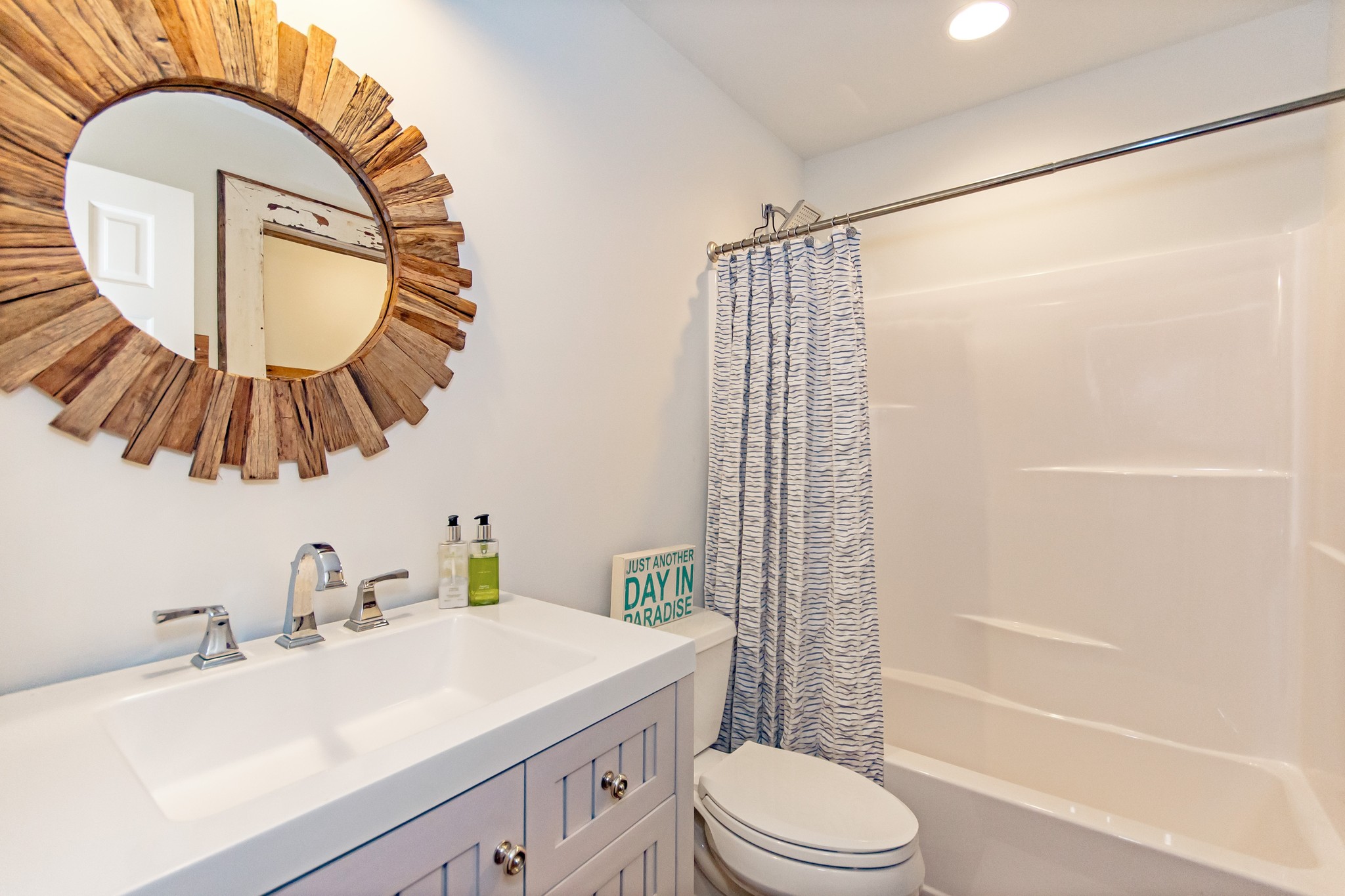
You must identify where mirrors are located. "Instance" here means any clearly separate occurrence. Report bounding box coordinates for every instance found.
[1,0,477,481]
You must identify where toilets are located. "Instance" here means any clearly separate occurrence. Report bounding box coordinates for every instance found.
[639,608,925,895]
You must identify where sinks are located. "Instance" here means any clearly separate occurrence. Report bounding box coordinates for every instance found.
[98,612,595,825]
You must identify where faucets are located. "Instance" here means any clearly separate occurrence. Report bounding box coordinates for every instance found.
[275,540,349,649]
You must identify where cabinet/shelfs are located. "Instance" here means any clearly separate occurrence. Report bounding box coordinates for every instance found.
[264,673,694,896]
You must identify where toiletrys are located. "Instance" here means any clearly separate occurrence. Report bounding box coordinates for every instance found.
[437,512,502,609]
